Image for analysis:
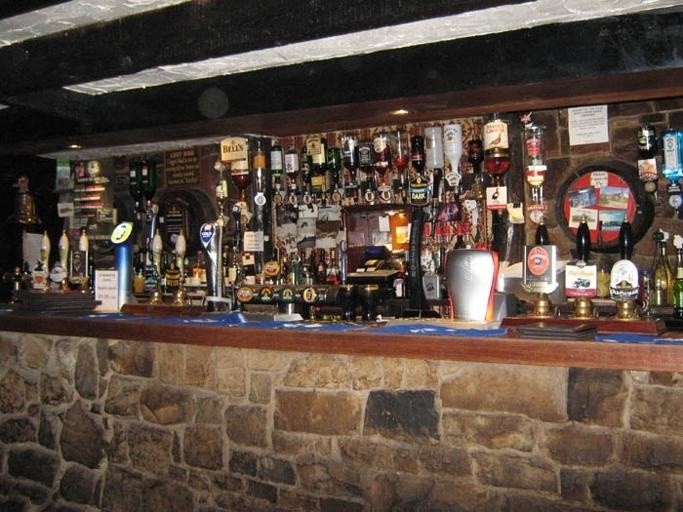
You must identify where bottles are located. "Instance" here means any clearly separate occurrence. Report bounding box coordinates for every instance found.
[128,161,155,212]
[618,211,633,264]
[216,112,548,216]
[9,260,46,290]
[134,228,338,303]
[576,214,591,265]
[651,231,682,308]
[78,229,88,276]
[58,230,68,270]
[664,126,682,188]
[534,216,549,246]
[636,123,661,192]
[39,229,51,275]
[426,233,473,271]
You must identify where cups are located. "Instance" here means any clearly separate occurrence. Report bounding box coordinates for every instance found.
[446,242,496,324]
[360,284,377,322]
[339,285,363,320]
